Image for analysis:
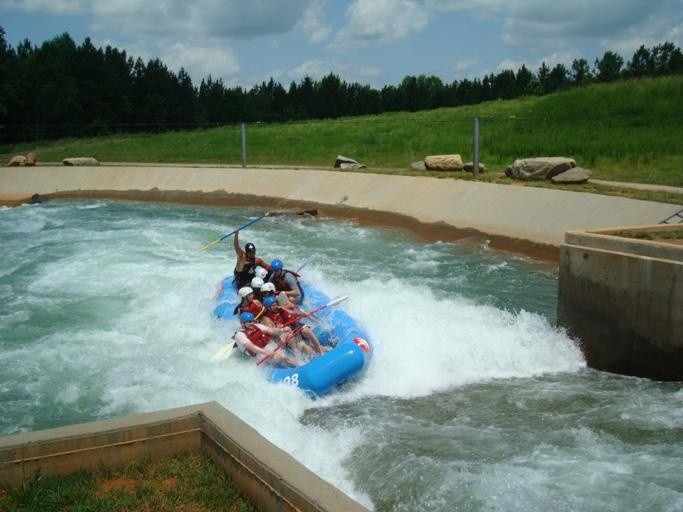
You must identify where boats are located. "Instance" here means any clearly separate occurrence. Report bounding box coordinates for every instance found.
[210,266,374,398]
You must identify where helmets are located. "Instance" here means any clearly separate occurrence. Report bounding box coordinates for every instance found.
[240,312,254,324]
[245,243,255,253]
[271,259,283,272]
[238,267,277,308]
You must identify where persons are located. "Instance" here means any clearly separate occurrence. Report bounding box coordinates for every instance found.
[232,229,334,368]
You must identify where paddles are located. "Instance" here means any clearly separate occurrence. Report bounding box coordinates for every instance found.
[214,306,265,360]
[278,295,348,329]
[198,212,271,252]
[296,253,317,275]
